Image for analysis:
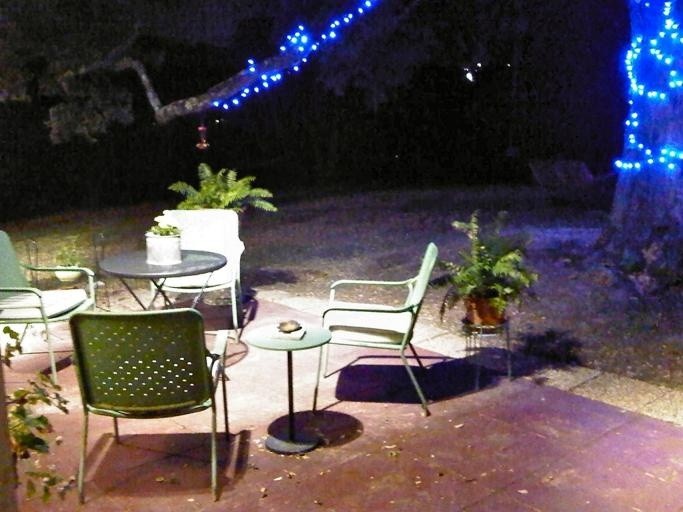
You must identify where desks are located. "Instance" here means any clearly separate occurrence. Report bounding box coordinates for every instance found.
[96,247,233,389]
[245,317,338,457]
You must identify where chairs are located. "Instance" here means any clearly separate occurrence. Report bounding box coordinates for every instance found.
[143,201,252,343]
[307,240,445,424]
[1,219,98,390]
[69,306,236,506]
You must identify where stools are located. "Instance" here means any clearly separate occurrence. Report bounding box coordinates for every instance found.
[457,316,515,395]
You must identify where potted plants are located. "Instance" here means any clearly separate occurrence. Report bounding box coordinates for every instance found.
[432,203,543,334]
[52,232,86,283]
[143,222,185,269]
